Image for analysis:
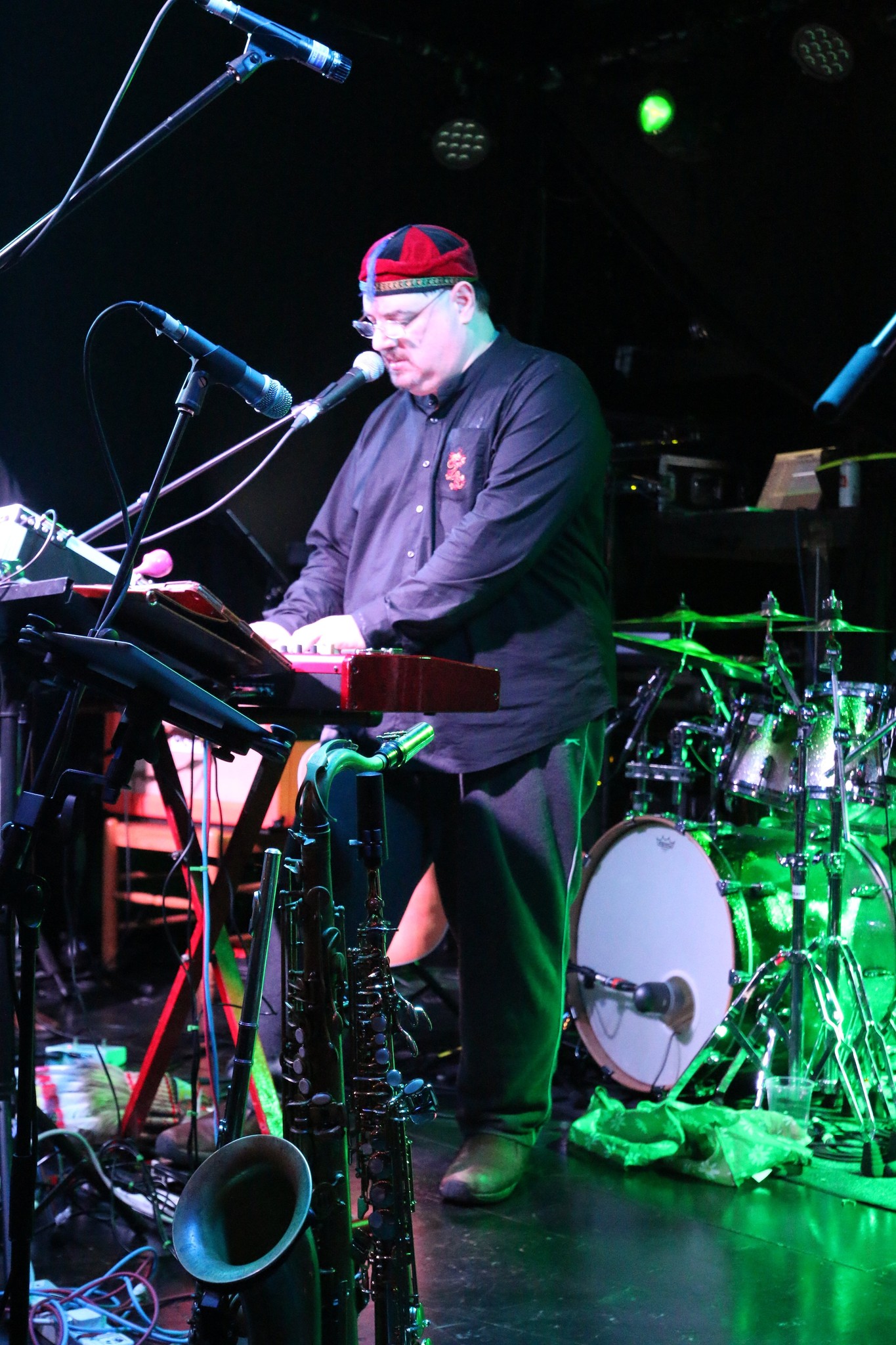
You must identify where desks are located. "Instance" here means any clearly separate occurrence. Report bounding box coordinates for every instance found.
[102,817,272,971]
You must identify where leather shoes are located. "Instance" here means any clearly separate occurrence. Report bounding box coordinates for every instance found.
[440,1131,531,1202]
[156,1097,263,1163]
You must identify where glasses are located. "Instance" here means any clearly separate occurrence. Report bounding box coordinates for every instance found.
[352,287,446,339]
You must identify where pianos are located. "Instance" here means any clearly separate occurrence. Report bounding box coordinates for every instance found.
[29,632,505,1153]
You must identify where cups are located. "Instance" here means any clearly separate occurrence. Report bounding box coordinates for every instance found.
[764,1076,815,1133]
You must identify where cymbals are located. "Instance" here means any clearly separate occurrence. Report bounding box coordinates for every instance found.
[614,607,892,634]
[612,631,769,685]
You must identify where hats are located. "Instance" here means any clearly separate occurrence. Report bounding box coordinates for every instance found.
[359,225,479,296]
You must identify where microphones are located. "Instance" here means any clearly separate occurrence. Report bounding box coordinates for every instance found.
[302,350,386,425]
[208,0,356,91]
[135,298,295,423]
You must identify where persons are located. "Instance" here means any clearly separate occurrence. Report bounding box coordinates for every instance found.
[157,224,617,1203]
[0,467,60,1040]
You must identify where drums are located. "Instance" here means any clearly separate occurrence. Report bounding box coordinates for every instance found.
[564,675,896,1108]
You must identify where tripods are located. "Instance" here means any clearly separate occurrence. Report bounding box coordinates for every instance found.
[658,652,895,1178]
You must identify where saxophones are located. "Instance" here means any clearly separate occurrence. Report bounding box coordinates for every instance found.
[158,723,439,1345]
